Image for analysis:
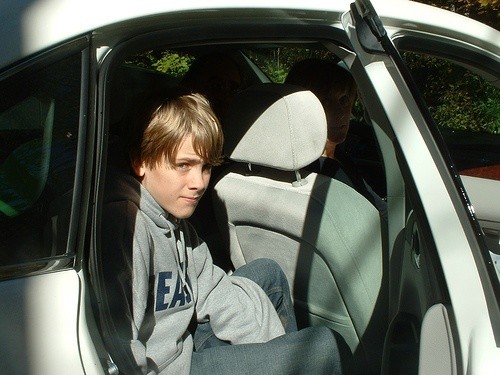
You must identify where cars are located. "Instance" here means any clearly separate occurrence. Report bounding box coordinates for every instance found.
[1,1,499,374]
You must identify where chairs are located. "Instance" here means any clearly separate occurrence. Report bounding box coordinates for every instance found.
[211,82,388,375]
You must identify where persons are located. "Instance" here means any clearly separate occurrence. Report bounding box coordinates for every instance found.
[97,86,348,375]
[284,58,388,206]
